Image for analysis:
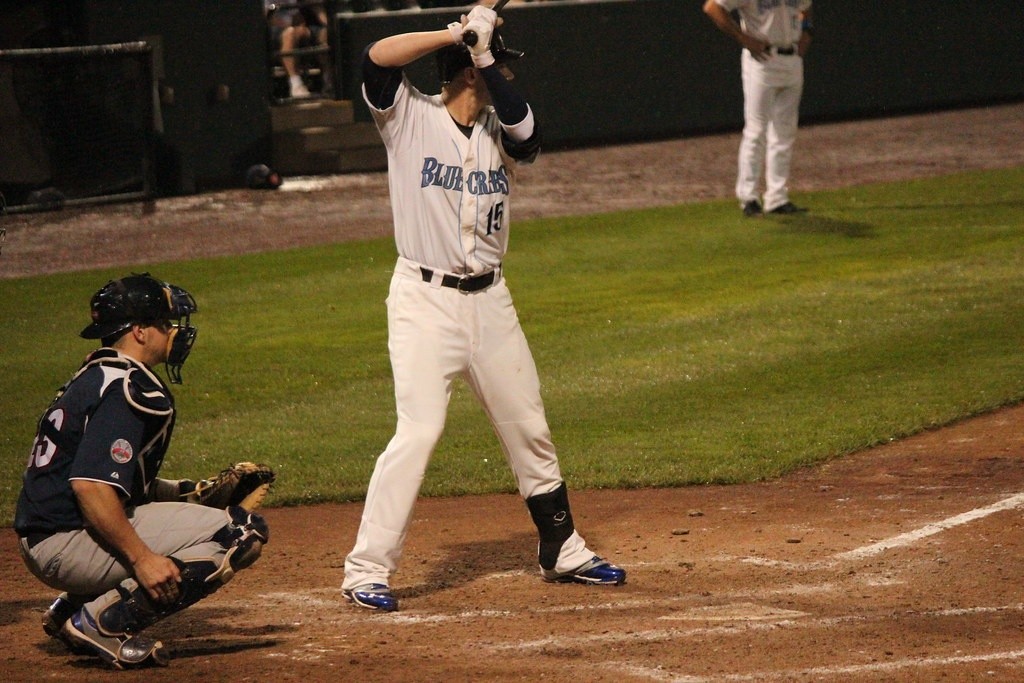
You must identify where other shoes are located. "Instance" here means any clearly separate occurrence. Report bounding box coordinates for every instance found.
[41,597,96,657]
[771,203,795,214]
[289,84,312,100]
[744,201,763,218]
[61,605,170,669]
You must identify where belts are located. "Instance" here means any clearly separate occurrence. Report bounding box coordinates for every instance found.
[421,263,501,294]
[766,46,795,56]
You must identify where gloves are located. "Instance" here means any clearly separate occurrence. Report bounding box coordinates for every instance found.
[464,6,497,68]
[446,12,503,45]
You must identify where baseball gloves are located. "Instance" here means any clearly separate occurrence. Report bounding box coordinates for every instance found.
[181,458,275,516]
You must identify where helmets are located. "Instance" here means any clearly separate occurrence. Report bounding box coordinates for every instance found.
[80,274,170,339]
[436,29,525,85]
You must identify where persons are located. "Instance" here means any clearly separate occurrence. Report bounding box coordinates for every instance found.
[14,274,273,664]
[264,0,328,99]
[703,0,813,218]
[342,6,626,612]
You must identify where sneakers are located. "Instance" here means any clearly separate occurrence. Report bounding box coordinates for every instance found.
[340,583,399,611]
[541,556,626,586]
